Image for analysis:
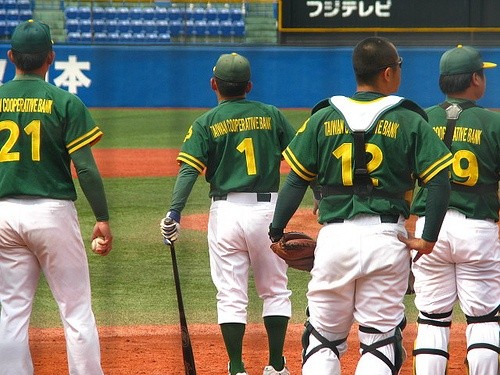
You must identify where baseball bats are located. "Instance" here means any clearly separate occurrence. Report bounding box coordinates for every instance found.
[164,217,196,375]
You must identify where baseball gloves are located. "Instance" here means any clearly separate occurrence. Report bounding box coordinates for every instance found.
[269,231,316,271]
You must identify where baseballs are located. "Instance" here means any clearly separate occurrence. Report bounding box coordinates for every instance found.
[90,238,105,255]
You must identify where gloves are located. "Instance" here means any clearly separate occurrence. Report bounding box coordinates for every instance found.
[160,210,181,245]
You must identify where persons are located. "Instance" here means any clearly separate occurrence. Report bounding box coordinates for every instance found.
[412,45,500,375]
[269,38,454,375]
[0,18,112,375]
[160,53,323,375]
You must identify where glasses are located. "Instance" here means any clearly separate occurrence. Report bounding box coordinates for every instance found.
[376,57,403,71]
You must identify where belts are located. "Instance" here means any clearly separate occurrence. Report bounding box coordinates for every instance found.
[214,192,272,202]
[326,214,400,223]
[419,213,486,220]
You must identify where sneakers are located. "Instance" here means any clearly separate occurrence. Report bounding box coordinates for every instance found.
[227,360,248,375]
[263,355,291,375]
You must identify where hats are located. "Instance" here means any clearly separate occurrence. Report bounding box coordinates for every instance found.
[12,19,54,54]
[212,53,250,82]
[439,44,497,75]
